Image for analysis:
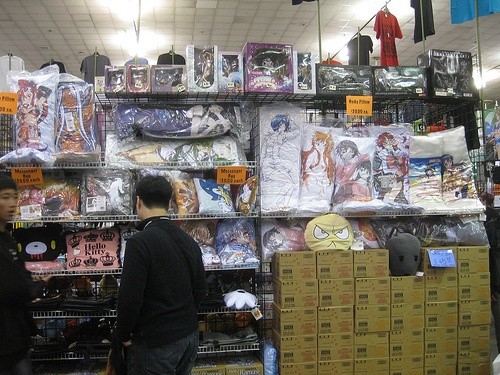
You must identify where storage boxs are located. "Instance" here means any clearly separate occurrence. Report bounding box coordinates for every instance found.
[191,355,264,375]
[105,42,473,96]
[270,245,492,375]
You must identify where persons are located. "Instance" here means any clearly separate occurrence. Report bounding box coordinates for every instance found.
[480,192,500,355]
[0,175,56,375]
[117,175,206,375]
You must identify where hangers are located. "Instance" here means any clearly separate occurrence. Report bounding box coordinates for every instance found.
[93,53,99,56]
[48,58,55,63]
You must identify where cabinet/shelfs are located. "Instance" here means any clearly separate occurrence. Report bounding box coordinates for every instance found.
[0,92,488,375]
